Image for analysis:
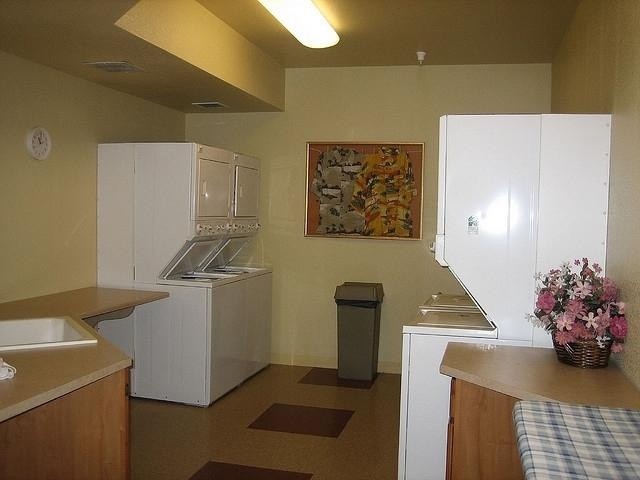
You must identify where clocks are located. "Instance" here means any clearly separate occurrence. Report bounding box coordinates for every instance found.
[27,126,52,160]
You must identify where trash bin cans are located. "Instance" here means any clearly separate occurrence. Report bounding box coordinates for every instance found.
[334,282,384,382]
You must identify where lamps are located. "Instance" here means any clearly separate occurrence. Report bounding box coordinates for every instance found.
[258,0,340,49]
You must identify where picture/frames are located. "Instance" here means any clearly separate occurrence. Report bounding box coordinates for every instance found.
[302,138,426,243]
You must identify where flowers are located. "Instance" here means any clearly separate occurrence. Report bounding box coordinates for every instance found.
[523,257,630,355]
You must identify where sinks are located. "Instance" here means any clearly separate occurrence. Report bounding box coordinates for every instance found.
[0,310,100,352]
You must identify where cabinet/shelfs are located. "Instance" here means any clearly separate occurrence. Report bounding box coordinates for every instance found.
[439,341,640,480]
[1,286,170,479]
[98,144,274,408]
